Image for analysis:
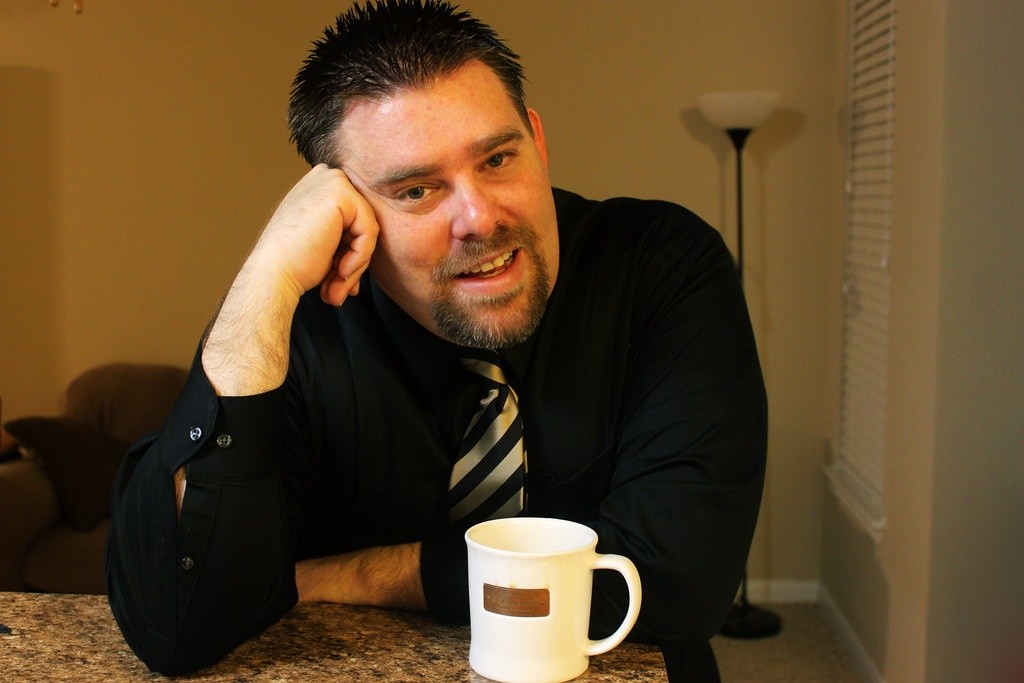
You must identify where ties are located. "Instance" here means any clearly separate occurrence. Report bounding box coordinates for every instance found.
[447,346,531,542]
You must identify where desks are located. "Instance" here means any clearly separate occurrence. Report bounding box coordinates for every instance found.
[0,592,669,683]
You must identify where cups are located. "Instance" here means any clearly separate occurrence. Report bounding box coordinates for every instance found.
[461,517,640,683]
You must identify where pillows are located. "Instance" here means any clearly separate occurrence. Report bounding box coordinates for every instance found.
[4,413,132,529]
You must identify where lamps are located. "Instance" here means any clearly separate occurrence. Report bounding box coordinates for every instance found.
[698,93,784,637]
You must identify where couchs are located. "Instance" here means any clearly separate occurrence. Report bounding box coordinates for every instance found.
[0,362,196,591]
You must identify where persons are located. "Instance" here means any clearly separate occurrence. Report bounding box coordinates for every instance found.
[106,0,770,678]
